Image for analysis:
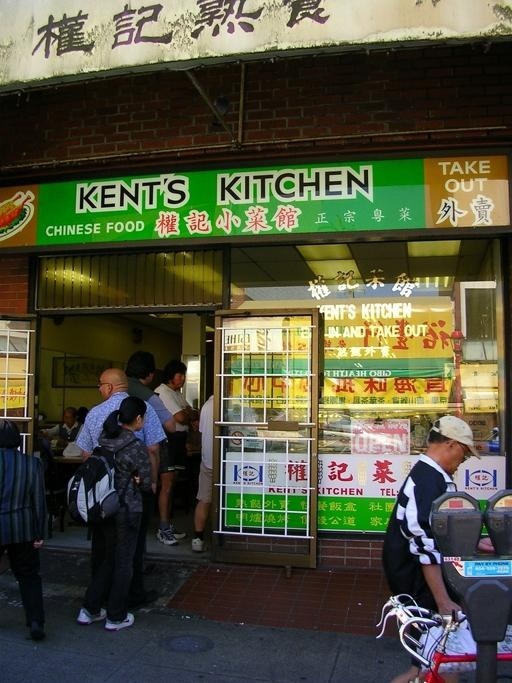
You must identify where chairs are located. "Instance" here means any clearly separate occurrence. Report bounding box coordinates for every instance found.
[40,455,67,539]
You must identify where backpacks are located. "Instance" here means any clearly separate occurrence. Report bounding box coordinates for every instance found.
[67,438,140,542]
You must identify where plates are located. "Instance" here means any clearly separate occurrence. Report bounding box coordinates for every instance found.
[1,201,34,244]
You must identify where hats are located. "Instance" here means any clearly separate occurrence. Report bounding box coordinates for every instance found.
[431,415,483,460]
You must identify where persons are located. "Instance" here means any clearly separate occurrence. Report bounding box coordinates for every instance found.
[191,395,214,551]
[0,419,46,641]
[77,395,152,631]
[75,368,168,609]
[382,416,493,683]
[151,359,200,546]
[40,406,84,442]
[125,350,176,434]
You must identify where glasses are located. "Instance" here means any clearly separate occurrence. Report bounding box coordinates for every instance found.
[456,441,472,460]
[96,381,110,387]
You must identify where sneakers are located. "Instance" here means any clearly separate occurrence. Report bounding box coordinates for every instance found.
[156,528,179,546]
[174,532,187,539]
[75,607,106,625]
[191,537,209,552]
[105,613,135,631]
